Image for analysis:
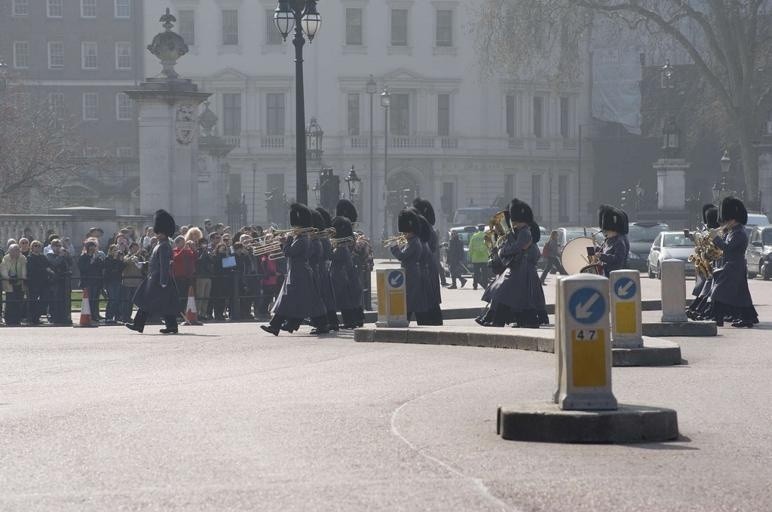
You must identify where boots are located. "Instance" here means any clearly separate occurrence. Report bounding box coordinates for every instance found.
[259,323,364,338]
[160,323,178,334]
[475,316,549,328]
[685,308,759,329]
[125,321,143,332]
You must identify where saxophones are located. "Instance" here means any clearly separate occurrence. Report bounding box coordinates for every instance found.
[688,224,729,280]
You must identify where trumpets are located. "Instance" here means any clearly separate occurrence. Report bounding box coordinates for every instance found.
[382,236,407,247]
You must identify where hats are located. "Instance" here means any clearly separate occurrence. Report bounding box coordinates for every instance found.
[509,198,533,221]
[719,197,747,224]
[599,205,629,234]
[703,204,713,224]
[398,209,418,233]
[332,217,353,238]
[311,207,331,229]
[414,198,435,224]
[418,214,431,241]
[154,209,176,238]
[706,207,720,228]
[336,198,357,222]
[530,222,540,243]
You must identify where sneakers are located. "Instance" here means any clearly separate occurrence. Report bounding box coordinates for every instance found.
[439,275,488,291]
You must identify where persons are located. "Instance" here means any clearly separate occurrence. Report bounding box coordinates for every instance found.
[260,201,374,337]
[540,231,569,286]
[1,224,159,328]
[447,231,467,289]
[593,204,630,277]
[169,219,286,326]
[685,196,759,328]
[126,210,177,334]
[389,198,448,325]
[468,198,550,328]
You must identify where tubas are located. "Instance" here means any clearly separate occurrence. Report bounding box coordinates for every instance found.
[483,210,515,273]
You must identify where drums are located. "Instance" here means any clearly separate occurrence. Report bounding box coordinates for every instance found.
[580,264,603,275]
[561,237,600,274]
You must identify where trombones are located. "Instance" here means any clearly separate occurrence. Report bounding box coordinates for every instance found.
[242,227,353,261]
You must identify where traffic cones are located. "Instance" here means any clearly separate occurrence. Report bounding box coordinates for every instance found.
[180,286,202,325]
[72,288,99,328]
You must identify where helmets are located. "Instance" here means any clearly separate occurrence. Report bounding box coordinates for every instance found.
[290,203,311,225]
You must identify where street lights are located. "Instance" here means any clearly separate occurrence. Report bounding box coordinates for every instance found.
[380,84,391,258]
[661,55,674,106]
[719,148,733,191]
[365,75,377,257]
[344,164,362,203]
[272,0,322,206]
